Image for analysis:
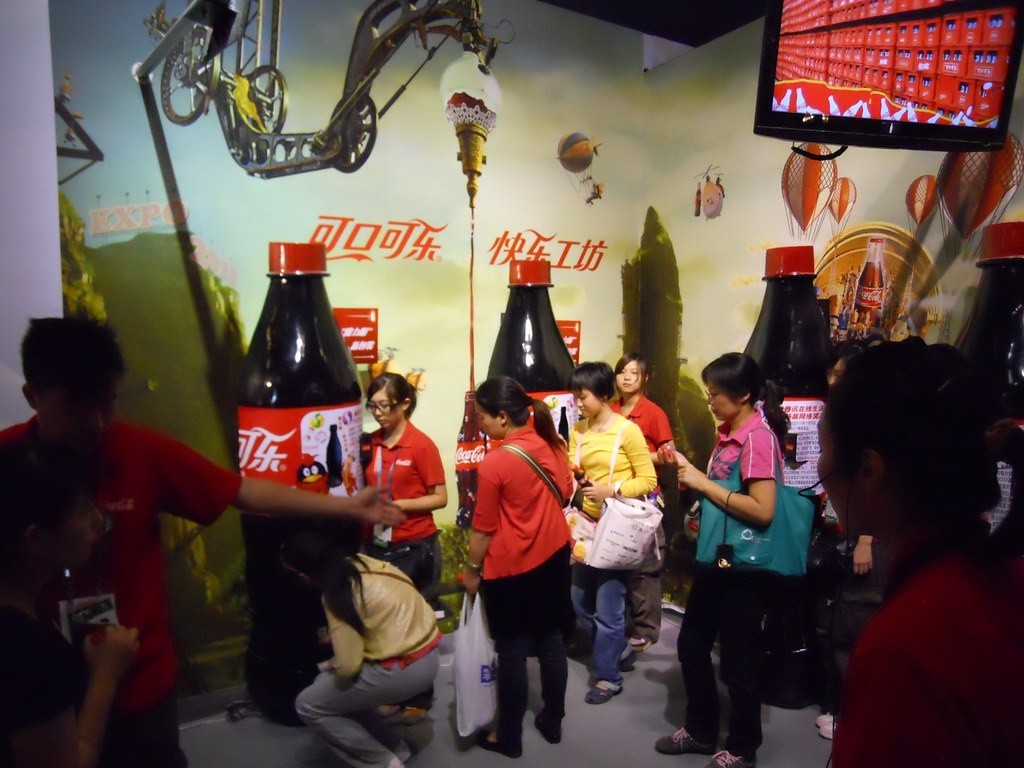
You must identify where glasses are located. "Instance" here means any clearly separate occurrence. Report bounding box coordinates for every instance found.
[364,400,407,414]
[706,388,734,402]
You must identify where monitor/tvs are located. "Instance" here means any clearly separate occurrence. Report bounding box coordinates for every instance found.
[750,0,1024,152]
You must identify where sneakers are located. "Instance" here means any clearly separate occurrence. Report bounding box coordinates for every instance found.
[703,749,758,768]
[655,725,716,755]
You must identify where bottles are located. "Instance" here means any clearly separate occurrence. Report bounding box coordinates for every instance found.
[489,258,604,656]
[569,460,599,502]
[375,349,391,379]
[404,369,423,396]
[848,238,884,342]
[952,222,1023,534]
[719,243,836,707]
[92,635,106,647]
[236,241,367,724]
[454,391,482,528]
[456,573,484,589]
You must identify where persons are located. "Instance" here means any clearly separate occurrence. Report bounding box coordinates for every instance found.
[570,361,664,705]
[362,373,449,609]
[0,315,409,768]
[457,375,574,758]
[276,523,443,768]
[0,416,139,768]
[614,352,677,652]
[654,354,819,768]
[817,340,1024,768]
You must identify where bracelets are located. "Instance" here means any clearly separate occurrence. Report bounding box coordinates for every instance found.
[467,559,482,571]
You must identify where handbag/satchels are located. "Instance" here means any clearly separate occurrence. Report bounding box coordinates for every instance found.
[451,587,498,736]
[695,428,817,575]
[585,493,662,572]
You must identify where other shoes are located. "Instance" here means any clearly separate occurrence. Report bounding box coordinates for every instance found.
[403,706,426,723]
[585,682,616,705]
[386,739,411,768]
[475,730,522,759]
[628,634,653,653]
[534,715,561,745]
[376,705,399,718]
[815,714,836,740]
